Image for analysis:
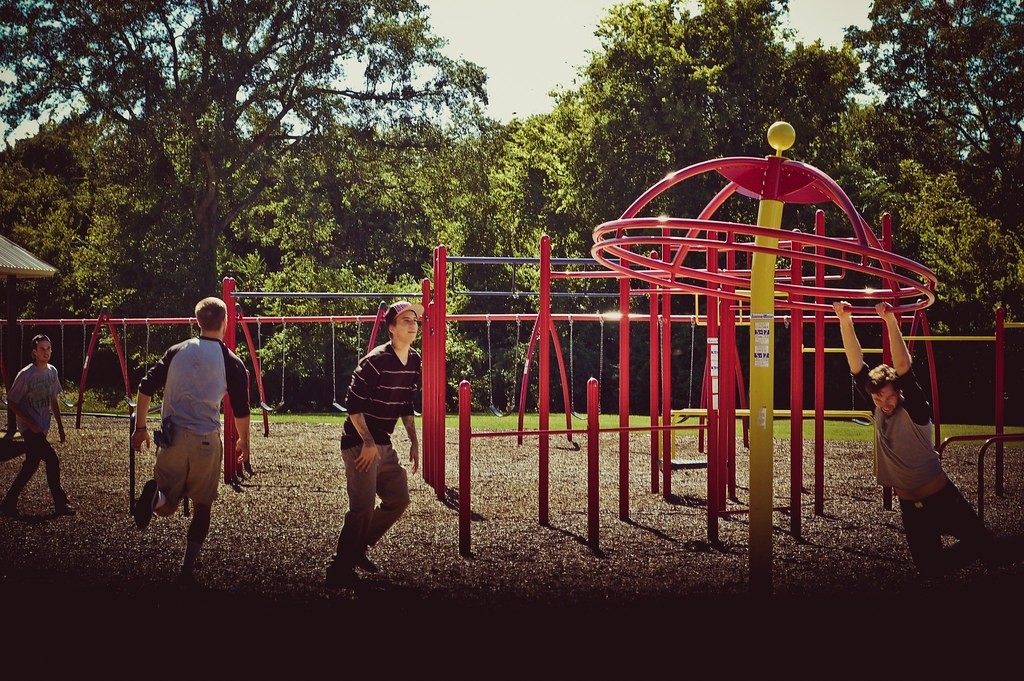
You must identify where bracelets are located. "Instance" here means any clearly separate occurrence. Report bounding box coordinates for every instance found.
[135,425,147,433]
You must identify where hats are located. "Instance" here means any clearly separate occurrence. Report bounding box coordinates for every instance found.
[385,301,424,325]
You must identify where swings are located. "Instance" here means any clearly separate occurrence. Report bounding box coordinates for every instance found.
[660,323,695,424]
[257,323,286,411]
[487,321,520,418]
[332,324,362,412]
[569,321,604,421]
[123,323,150,408]
[851,378,874,427]
[59,324,87,408]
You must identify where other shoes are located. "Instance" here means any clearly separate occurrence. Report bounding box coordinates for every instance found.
[354,549,378,572]
[56,503,76,516]
[0,504,17,515]
[326,563,361,585]
[134,480,160,529]
[179,570,197,586]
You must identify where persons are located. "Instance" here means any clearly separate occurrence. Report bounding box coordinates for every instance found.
[325,301,424,589]
[129,297,251,582]
[0,334,76,517]
[832,300,1024,580]
